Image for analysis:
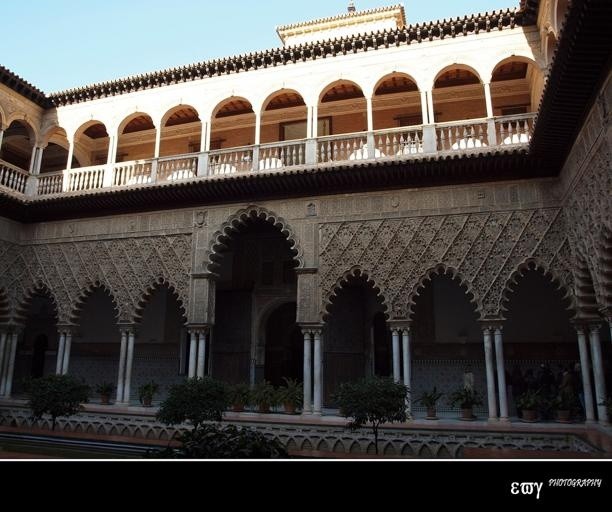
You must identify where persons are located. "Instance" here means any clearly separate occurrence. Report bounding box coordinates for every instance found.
[504,361,586,421]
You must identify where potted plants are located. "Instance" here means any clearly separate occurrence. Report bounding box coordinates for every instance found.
[446,383,484,421]
[328,382,361,417]
[135,379,159,407]
[411,386,443,420]
[515,388,578,423]
[223,376,303,414]
[78,380,117,404]
